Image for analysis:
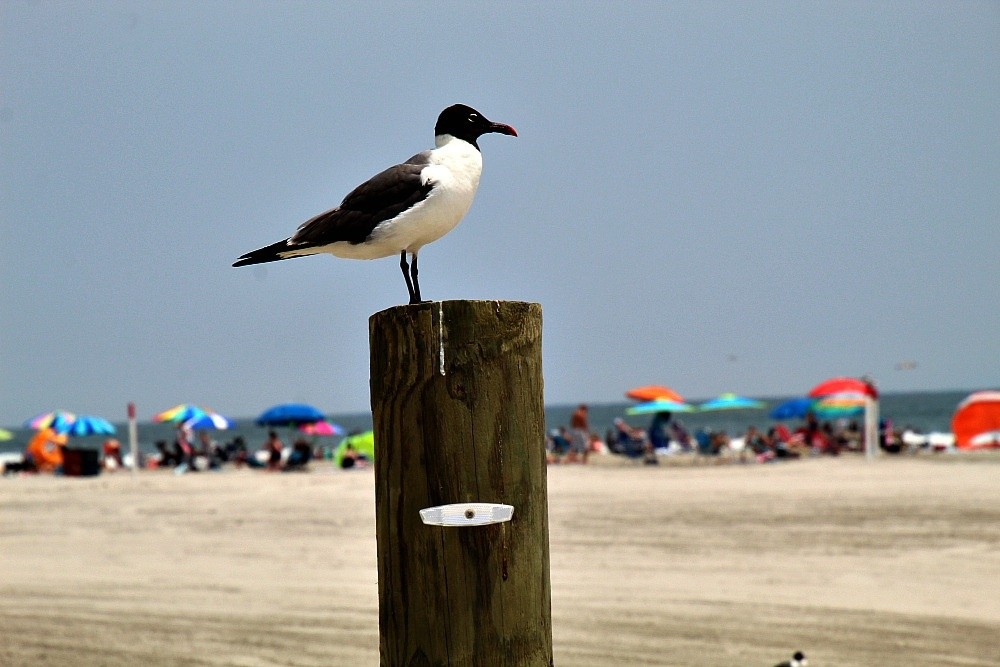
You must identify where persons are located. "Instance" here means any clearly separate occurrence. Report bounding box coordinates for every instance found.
[341,443,366,468]
[568,404,590,464]
[155,429,314,465]
[545,418,864,463]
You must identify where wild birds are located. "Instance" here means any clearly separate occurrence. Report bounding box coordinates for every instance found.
[228,102,520,309]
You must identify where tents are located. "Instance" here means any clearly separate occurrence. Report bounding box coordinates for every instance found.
[334,430,374,464]
[951,389,1000,452]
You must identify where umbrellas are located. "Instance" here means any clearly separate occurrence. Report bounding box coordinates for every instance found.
[770,397,823,420]
[60,415,118,437]
[812,391,873,420]
[182,412,238,430]
[625,397,697,415]
[625,384,683,403]
[23,408,75,433]
[298,418,344,436]
[152,402,213,422]
[254,402,324,428]
[808,378,878,399]
[695,392,768,412]
